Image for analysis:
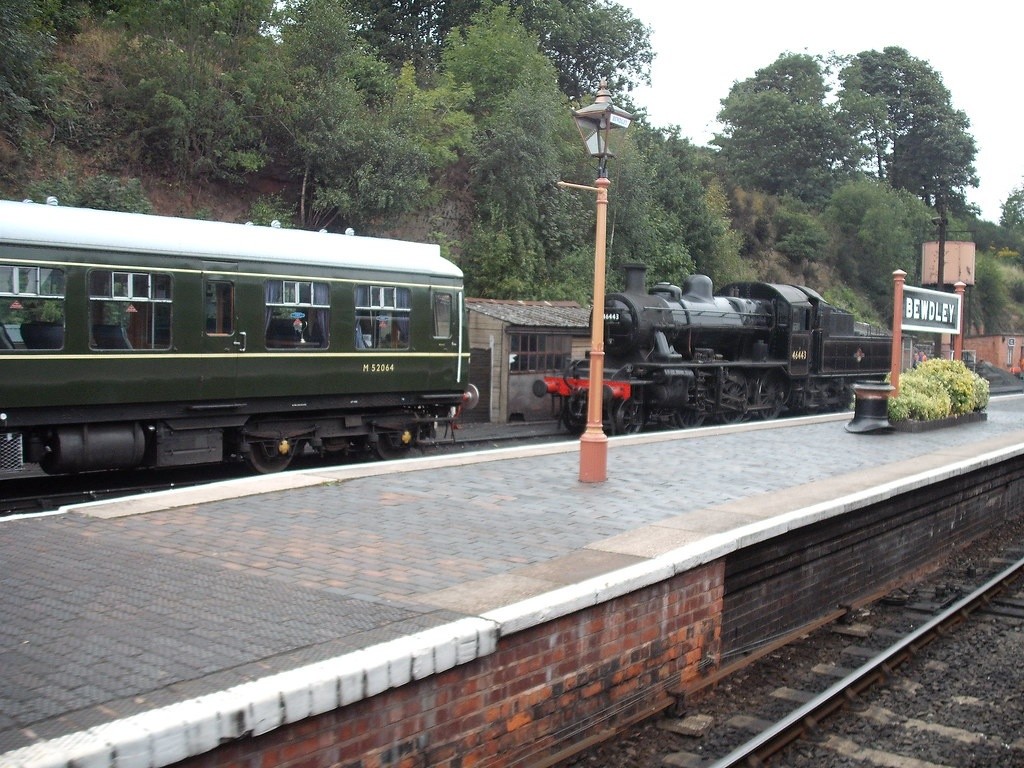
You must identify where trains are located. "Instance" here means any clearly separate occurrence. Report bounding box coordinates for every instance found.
[543,263,894,435]
[0,185,481,480]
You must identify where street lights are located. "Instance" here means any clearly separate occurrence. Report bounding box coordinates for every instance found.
[568,76,635,485]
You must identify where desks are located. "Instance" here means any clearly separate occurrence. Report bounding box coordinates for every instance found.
[266,338,320,348]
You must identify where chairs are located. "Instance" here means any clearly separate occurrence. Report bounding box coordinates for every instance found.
[0,323,16,349]
[91,325,135,350]
[20,321,64,349]
